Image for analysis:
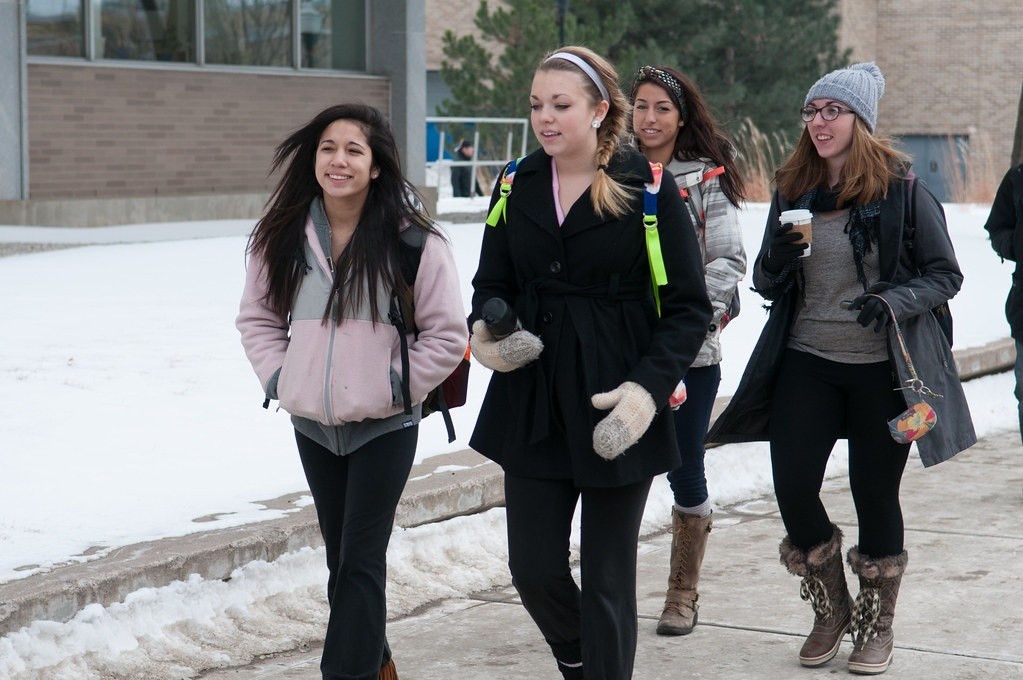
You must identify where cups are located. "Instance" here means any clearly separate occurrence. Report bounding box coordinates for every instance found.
[779,208,814,258]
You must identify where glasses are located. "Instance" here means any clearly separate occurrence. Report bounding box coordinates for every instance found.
[800,105,856,122]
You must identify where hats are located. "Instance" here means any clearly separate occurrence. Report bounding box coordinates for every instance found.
[803,62,886,133]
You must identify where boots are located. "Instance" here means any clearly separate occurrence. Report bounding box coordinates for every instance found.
[847,546,908,673]
[657,506,714,633]
[778,523,856,665]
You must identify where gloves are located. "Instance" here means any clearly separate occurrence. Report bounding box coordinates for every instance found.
[469,319,543,372]
[761,223,809,276]
[848,295,890,333]
[592,382,657,460]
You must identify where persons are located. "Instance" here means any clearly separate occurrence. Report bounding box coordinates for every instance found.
[628,65,747,635]
[703,62,978,674]
[984,162,1023,439]
[468,45,714,680]
[450,141,484,198]
[235,103,469,680]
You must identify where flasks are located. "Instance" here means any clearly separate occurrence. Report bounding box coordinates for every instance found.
[481,297,538,371]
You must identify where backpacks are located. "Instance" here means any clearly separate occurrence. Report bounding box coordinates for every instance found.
[279,211,469,418]
